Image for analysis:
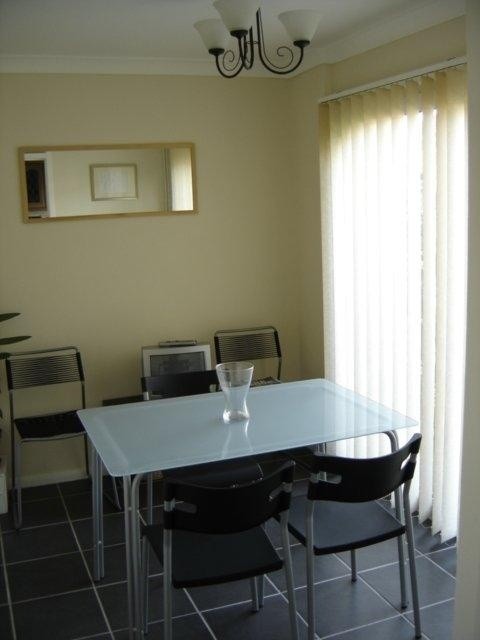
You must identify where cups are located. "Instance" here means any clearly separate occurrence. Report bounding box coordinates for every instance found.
[220,417,253,458]
[216,362,255,419]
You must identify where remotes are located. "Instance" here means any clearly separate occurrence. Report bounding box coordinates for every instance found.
[159,340,197,346]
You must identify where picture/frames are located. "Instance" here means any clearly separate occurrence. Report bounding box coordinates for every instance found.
[88,162,139,202]
[25,160,47,212]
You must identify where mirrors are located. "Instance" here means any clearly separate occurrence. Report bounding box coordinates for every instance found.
[17,143,200,225]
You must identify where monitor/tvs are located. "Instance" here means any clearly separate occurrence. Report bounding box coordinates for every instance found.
[142,342,212,401]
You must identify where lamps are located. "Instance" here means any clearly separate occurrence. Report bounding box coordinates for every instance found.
[193,0,326,78]
